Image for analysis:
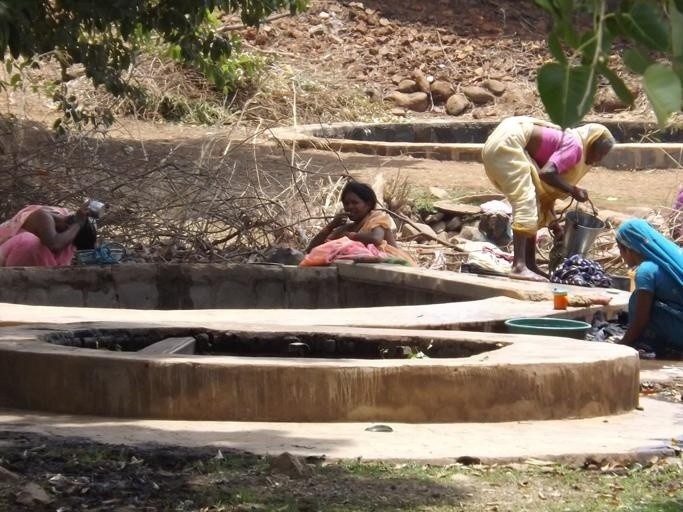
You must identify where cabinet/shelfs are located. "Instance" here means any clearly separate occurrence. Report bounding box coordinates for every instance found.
[552,290,568,310]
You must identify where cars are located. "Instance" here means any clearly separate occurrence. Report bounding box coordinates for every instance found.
[87,200,106,219]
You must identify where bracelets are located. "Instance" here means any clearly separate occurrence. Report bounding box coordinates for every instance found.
[504,317,592,339]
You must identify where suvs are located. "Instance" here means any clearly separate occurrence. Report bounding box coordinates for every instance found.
[561,201,606,253]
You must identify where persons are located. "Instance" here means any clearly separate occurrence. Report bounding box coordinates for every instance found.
[305,183,397,258]
[615,219,682,357]
[482,115,616,283]
[1,199,93,267]
